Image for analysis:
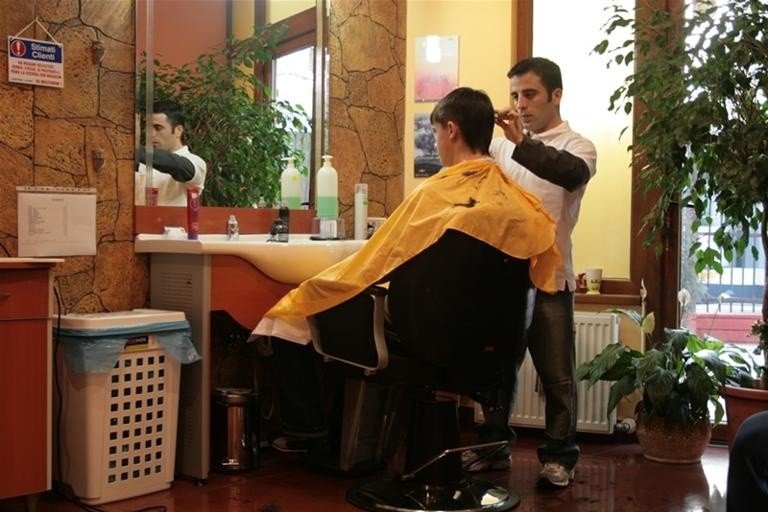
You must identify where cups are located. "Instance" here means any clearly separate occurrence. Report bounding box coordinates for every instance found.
[578,268,603,295]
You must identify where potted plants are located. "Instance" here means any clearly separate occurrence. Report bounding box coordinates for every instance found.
[576,277,751,465]
[589,0,768,453]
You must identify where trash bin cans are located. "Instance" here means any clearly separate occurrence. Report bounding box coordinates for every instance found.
[52,308,191,507]
[210,387,259,472]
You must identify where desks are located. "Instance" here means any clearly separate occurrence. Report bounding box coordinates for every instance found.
[133,234,369,490]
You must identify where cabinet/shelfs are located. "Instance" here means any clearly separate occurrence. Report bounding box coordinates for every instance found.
[1,257,67,511]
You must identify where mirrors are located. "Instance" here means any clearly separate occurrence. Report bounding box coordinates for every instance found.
[134,0,323,234]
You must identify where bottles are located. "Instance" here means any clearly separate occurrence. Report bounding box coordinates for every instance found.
[226,215,240,241]
[352,183,369,240]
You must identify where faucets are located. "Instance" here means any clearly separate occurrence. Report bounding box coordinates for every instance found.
[265,219,284,241]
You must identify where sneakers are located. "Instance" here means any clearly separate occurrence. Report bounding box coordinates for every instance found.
[461,446,512,472]
[271,428,333,452]
[535,462,575,488]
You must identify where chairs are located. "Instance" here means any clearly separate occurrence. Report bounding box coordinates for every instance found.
[305,227,531,511]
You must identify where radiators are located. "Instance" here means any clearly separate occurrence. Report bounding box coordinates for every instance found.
[473,311,637,436]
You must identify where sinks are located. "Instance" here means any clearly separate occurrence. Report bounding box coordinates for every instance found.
[239,235,370,285]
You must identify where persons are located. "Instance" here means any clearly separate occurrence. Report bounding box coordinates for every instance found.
[488,56,598,489]
[136,98,207,207]
[244,86,565,452]
